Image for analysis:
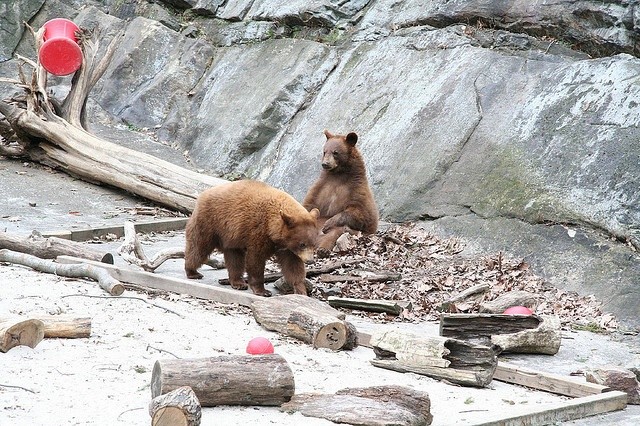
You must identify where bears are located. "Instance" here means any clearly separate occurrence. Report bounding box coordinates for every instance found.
[302,129,378,258]
[185,179,321,297]
